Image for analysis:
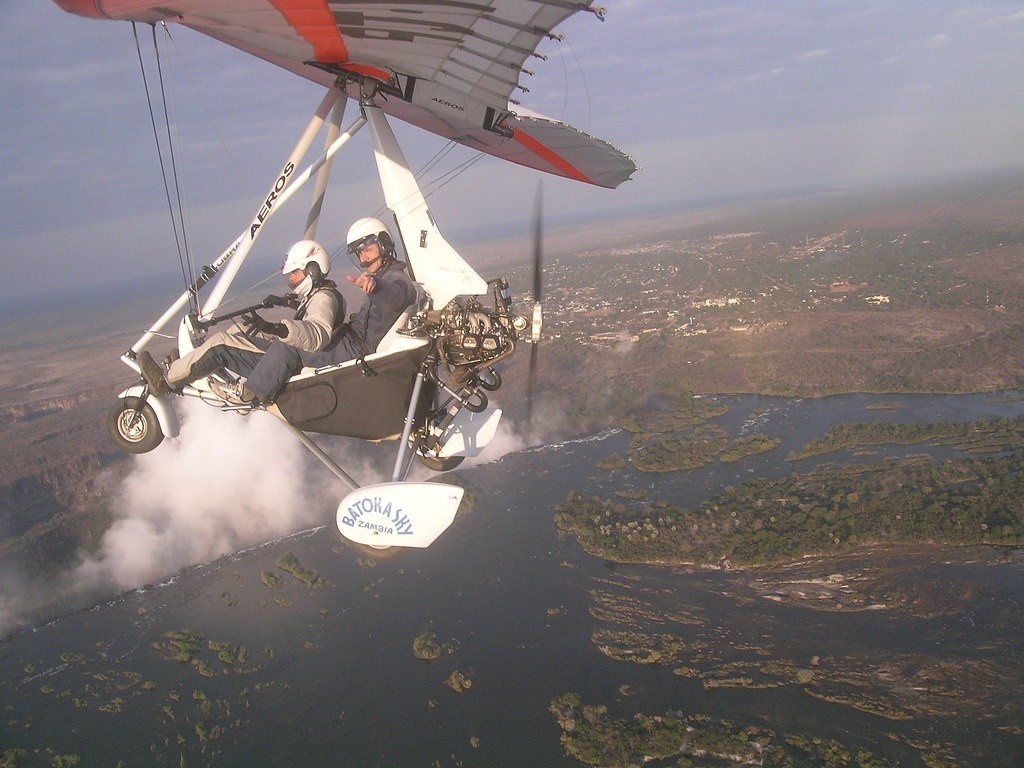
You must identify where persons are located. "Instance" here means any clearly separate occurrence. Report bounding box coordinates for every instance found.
[207,217,416,416]
[138,239,347,397]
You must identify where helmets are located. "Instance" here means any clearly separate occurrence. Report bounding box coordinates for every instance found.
[347,217,394,245]
[283,240,331,274]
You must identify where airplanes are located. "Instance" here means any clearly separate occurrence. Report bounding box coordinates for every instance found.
[54,0,635,560]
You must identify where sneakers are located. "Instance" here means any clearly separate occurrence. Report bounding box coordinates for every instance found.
[207,376,253,415]
[138,351,173,397]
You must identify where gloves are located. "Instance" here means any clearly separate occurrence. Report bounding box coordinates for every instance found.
[263,295,288,308]
[241,309,288,338]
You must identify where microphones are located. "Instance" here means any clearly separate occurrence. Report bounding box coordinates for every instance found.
[360,254,383,268]
